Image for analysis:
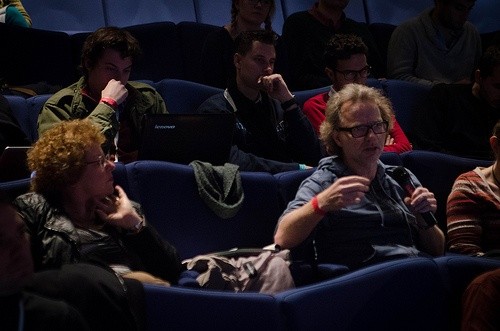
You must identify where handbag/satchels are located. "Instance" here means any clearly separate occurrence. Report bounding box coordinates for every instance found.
[180,244,295,294]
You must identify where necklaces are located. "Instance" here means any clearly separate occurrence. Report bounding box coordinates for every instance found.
[492,168,500,185]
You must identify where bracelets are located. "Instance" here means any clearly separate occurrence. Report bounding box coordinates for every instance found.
[417,224,429,232]
[100,97,119,108]
[281,95,296,110]
[312,196,326,216]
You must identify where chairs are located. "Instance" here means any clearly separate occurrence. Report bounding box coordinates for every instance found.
[0,20,500,331]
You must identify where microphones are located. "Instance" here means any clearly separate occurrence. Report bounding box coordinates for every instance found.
[393,167,438,228]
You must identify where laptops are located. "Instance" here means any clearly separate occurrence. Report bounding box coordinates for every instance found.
[136,112,233,165]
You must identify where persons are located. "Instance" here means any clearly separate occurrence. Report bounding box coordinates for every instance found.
[37,26,168,158]
[446,120,500,256]
[190,0,483,88]
[273,84,445,273]
[0,118,188,331]
[0,0,32,28]
[197,29,317,174]
[418,30,500,161]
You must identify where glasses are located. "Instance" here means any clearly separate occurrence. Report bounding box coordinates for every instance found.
[334,117,390,138]
[245,0,274,7]
[82,153,112,168]
[333,63,373,81]
